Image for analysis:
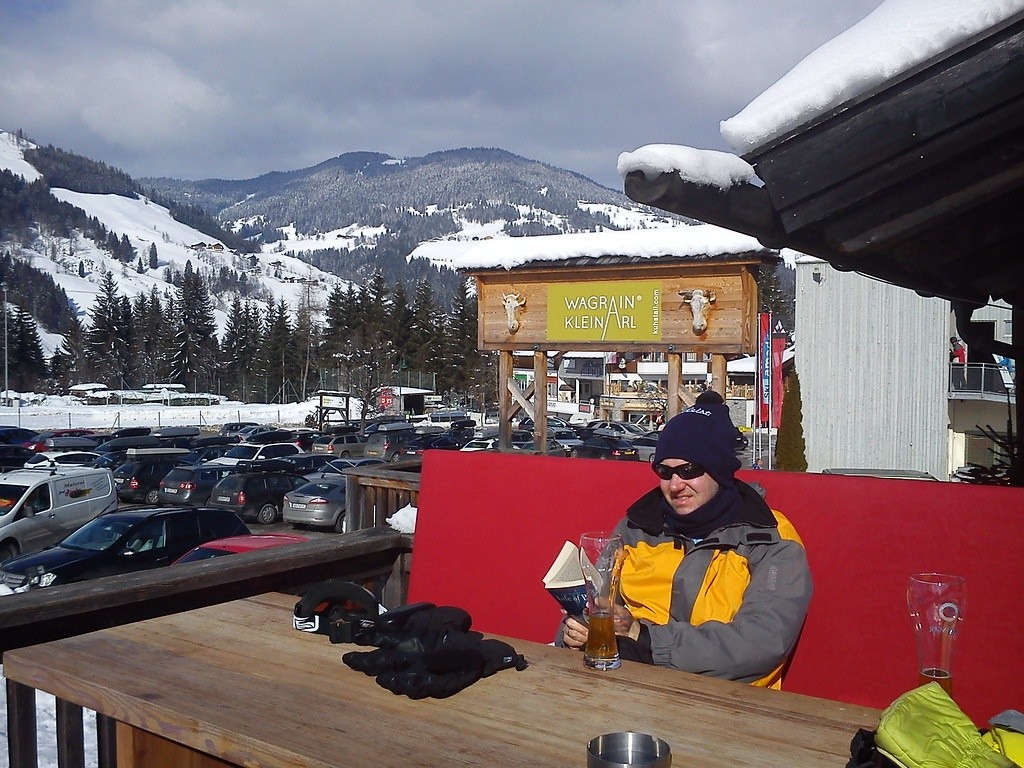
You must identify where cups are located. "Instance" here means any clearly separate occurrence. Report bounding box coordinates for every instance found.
[579,532,624,672]
[906,571,967,697]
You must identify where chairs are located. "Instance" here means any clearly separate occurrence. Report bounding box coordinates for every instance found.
[126,539,142,553]
[138,535,164,551]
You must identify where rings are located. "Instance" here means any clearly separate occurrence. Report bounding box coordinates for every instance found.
[568,629,572,638]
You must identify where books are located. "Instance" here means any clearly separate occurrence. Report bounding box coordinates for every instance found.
[542,540,604,617]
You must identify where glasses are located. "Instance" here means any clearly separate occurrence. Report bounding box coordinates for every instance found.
[655,460,706,479]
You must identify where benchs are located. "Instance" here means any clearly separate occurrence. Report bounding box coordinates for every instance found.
[408,448,1023,734]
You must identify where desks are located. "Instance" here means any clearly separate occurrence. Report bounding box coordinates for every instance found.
[3,592,885,768]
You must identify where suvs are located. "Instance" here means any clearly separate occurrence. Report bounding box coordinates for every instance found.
[206,473,312,525]
[312,435,367,460]
[159,466,256,510]
[0,506,255,594]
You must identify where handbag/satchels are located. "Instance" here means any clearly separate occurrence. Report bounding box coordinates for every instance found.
[293,580,387,634]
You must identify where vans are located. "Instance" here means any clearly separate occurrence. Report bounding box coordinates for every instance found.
[0,462,118,570]
[363,433,421,462]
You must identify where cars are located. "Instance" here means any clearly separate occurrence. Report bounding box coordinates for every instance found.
[283,479,348,534]
[403,435,464,462]
[217,418,328,453]
[461,437,521,455]
[510,415,663,464]
[0,420,219,505]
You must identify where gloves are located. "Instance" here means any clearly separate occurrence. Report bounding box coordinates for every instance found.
[342,608,519,699]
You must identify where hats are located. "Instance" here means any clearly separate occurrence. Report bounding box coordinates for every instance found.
[652,391,742,488]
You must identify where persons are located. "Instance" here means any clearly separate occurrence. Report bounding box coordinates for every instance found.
[950,335,967,390]
[752,459,764,470]
[555,391,816,694]
